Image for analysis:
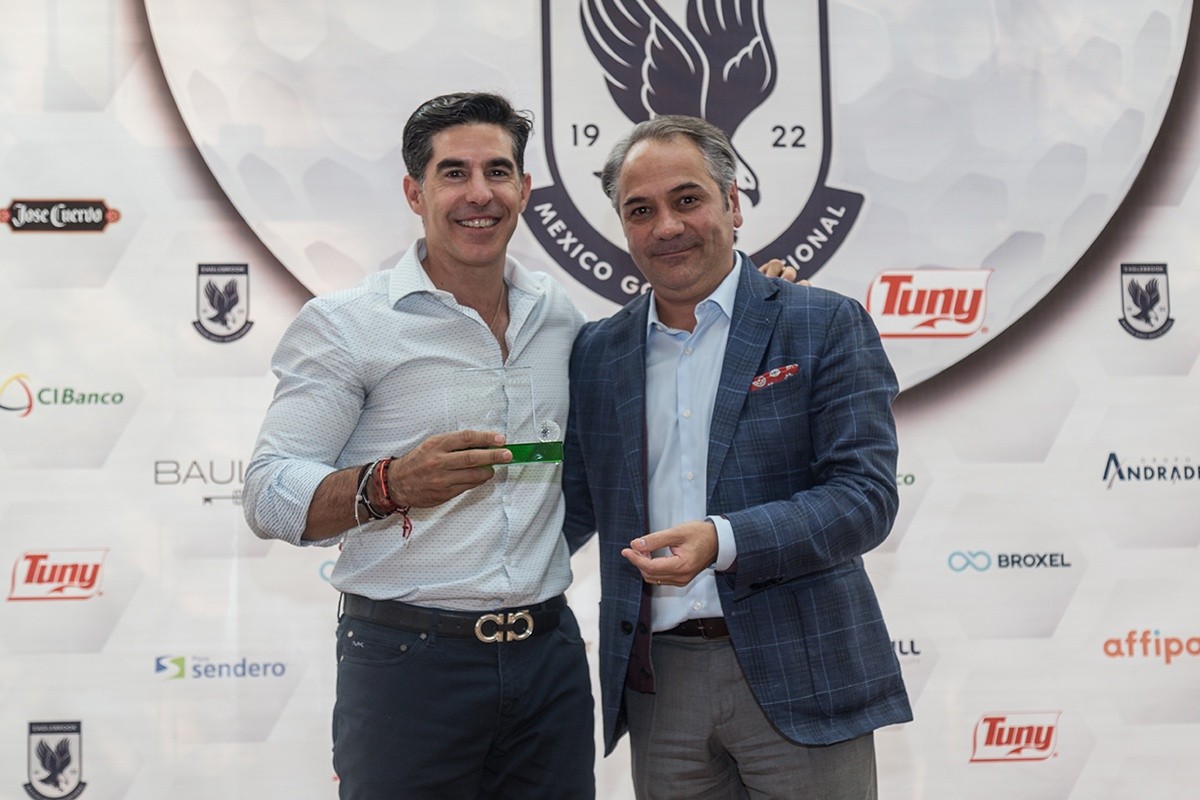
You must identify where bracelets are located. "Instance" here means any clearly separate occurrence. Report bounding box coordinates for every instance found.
[354,457,412,540]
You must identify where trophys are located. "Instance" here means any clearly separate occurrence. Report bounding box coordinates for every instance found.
[455,368,564,466]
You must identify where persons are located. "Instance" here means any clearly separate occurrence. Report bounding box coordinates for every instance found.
[562,115,914,800]
[242,94,811,800]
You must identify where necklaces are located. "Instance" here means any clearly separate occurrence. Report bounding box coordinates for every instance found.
[488,283,505,330]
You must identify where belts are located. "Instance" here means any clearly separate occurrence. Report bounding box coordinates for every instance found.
[656,617,729,641]
[343,592,568,643]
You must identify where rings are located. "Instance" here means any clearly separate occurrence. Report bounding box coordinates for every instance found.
[657,579,662,586]
